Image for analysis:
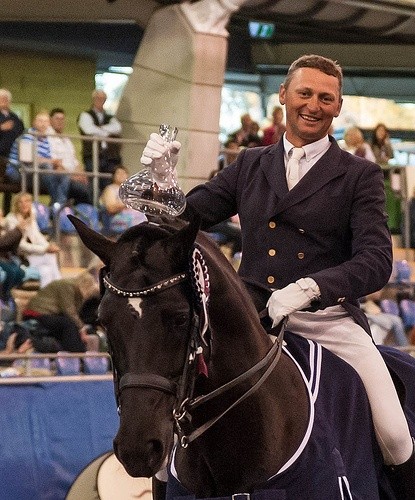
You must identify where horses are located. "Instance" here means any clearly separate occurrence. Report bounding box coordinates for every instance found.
[65,209,314,500]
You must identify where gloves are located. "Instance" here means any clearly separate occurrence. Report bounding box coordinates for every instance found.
[140,132,183,176]
[266,277,321,329]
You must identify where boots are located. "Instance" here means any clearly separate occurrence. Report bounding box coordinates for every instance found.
[380,438,415,500]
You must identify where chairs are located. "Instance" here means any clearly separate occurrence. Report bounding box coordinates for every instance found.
[0,203,415,375]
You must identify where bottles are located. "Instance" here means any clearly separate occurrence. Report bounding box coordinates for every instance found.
[118,123,187,218]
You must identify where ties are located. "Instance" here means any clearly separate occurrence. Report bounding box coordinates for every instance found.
[286,146,305,194]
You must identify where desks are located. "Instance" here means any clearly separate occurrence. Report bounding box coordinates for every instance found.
[380,164,415,247]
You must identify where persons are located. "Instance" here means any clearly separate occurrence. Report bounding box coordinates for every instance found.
[140,55,415,500]
[0,88,415,367]
